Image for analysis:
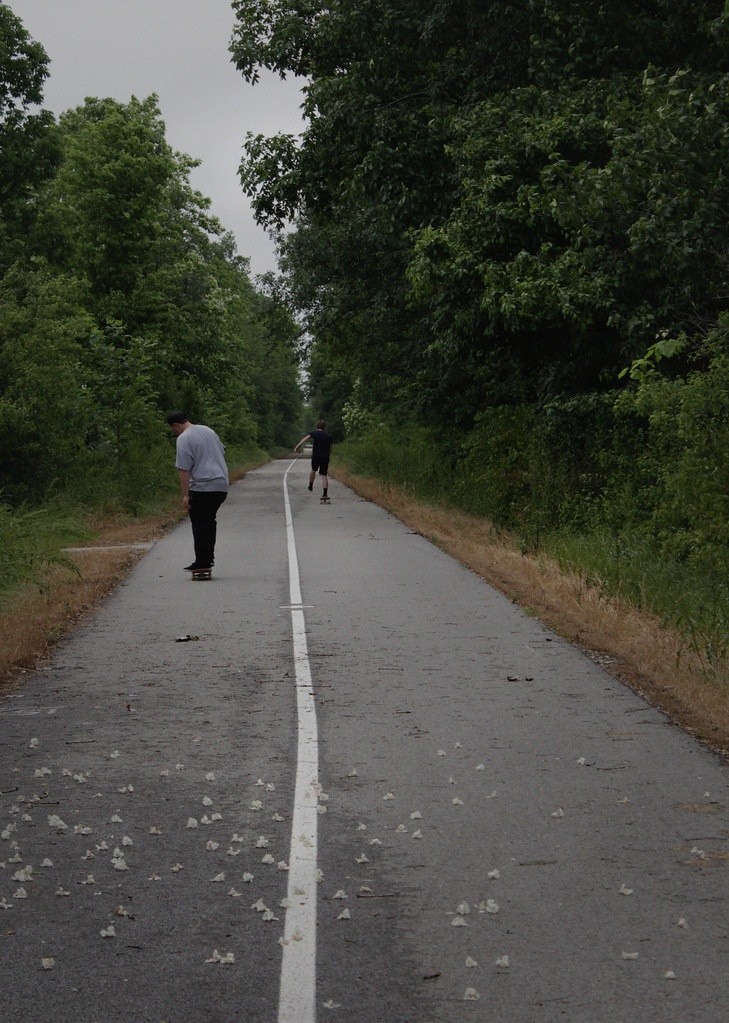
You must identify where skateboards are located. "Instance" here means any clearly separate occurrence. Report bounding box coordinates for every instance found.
[320,497,330,504]
[192,568,212,581]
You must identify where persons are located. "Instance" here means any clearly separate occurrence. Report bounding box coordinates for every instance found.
[165,410,229,572]
[294,420,332,498]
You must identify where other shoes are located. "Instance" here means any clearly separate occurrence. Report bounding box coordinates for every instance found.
[322,494,327,497]
[184,559,214,570]
[307,487,313,491]
